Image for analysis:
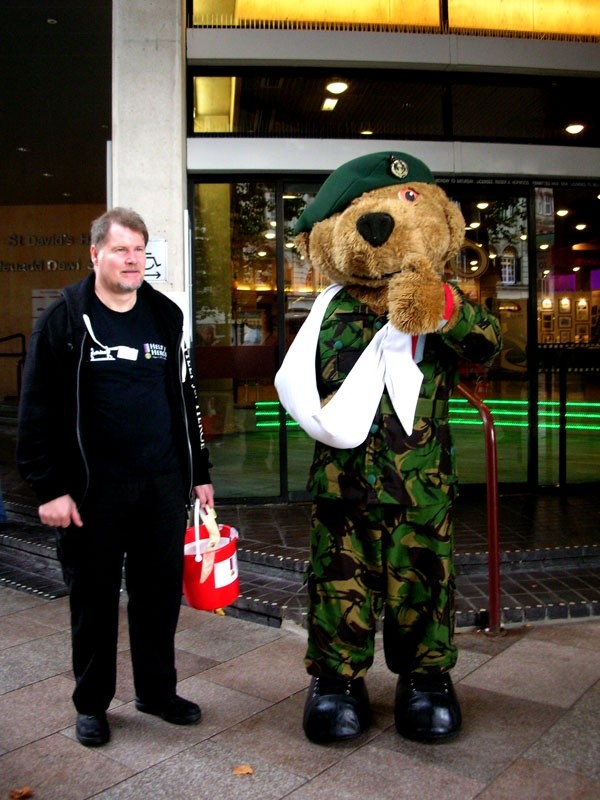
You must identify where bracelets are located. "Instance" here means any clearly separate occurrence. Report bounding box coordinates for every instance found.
[434,278,456,331]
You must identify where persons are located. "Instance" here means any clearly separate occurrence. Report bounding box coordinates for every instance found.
[16,210,216,747]
[255,243,291,347]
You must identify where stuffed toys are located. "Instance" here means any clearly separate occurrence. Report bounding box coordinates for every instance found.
[271,147,504,746]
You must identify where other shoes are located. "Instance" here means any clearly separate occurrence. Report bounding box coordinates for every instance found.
[75,710,110,746]
[134,694,201,724]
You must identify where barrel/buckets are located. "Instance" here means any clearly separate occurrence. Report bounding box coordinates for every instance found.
[183,499,243,612]
